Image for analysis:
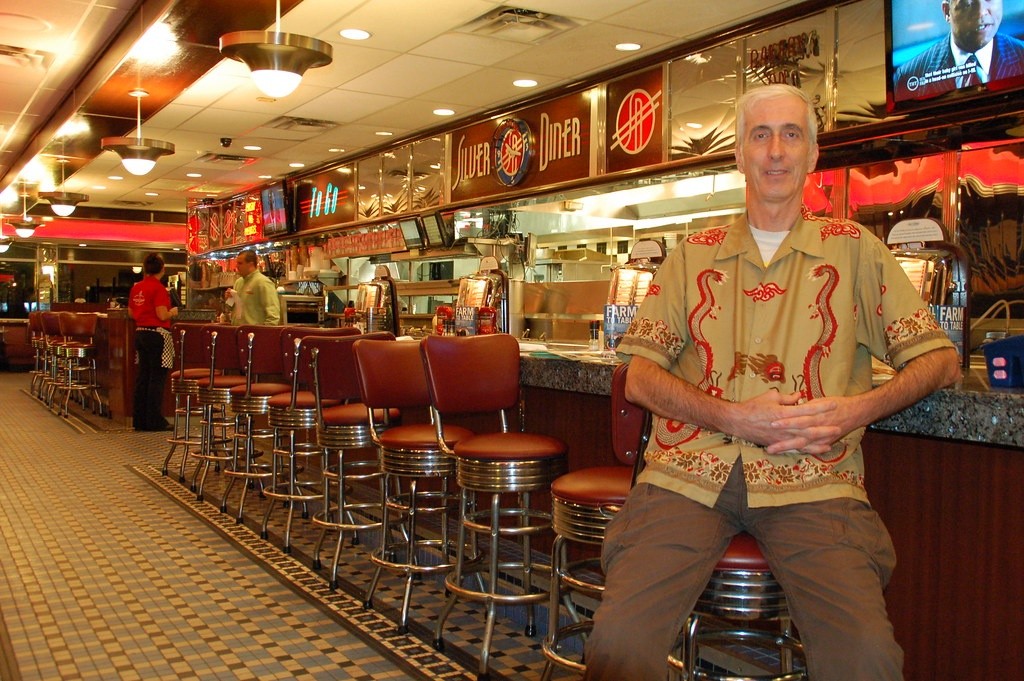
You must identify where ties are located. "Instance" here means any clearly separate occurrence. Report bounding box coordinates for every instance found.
[960,55,982,88]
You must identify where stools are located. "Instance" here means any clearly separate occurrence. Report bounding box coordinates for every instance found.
[260,324,363,550]
[350,337,491,634]
[418,332,590,681]
[666,531,809,681]
[296,333,422,592]
[189,323,264,502]
[160,322,243,484]
[31,311,104,418]
[219,323,322,524]
[538,358,653,681]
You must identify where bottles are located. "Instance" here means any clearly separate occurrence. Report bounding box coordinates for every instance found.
[442,313,455,336]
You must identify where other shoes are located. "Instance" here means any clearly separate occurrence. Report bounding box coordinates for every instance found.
[134,424,174,430]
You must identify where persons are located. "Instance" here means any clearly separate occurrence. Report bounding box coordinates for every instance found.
[894,0,1024,100]
[224,250,281,326]
[585,85,959,681]
[129,254,179,431]
[265,190,282,232]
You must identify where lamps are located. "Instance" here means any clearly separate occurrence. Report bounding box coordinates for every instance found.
[0,216,17,253]
[37,159,90,217]
[98,88,177,176]
[8,194,41,238]
[218,0,334,97]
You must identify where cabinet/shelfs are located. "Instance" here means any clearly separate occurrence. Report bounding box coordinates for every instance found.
[397,279,461,336]
[323,284,359,325]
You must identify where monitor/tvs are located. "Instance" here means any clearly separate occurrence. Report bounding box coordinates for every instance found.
[883,0,1024,116]
[259,179,291,238]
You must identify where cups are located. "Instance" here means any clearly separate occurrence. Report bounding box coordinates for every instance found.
[589,320,600,350]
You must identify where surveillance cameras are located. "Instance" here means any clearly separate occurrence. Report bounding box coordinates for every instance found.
[220,138,232,147]
